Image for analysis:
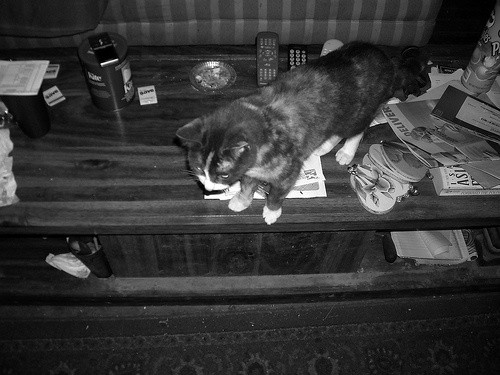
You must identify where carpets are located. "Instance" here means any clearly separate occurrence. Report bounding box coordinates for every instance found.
[0,294,500,375]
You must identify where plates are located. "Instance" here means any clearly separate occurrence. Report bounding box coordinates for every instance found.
[189,61,238,93]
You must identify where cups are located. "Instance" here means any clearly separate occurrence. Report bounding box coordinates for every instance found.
[0,88,52,139]
[66,239,112,278]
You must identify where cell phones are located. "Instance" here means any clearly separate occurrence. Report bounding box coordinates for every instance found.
[87,31,120,67]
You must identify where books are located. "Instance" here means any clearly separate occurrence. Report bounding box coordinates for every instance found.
[381,67,499,266]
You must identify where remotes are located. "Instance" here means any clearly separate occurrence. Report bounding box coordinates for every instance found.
[256,32,279,86]
[287,43,307,71]
[319,39,344,58]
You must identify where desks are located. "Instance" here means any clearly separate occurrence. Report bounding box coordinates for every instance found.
[0,44,500,306]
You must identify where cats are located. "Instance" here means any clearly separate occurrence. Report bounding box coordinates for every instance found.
[175,40,454,225]
